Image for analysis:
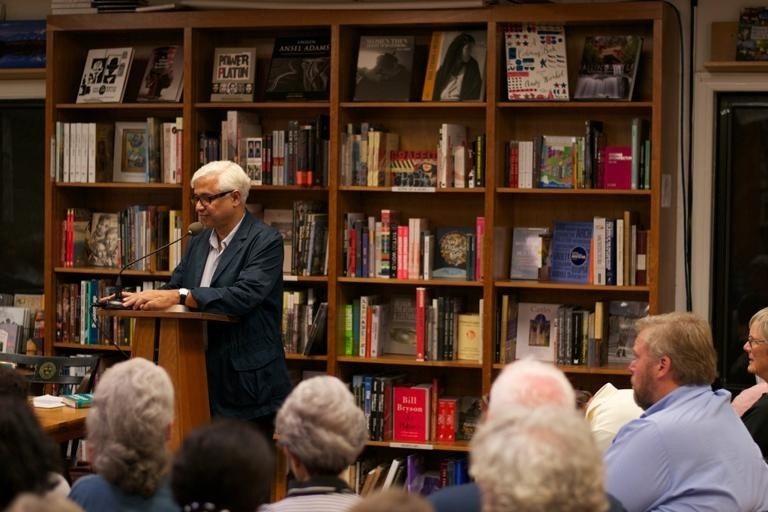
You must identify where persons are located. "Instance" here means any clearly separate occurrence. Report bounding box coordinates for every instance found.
[260,376,368,512]
[65,357,183,512]
[469,405,610,511]
[604,313,768,512]
[730,306,768,465]
[171,419,274,512]
[425,359,627,512]
[98,159,292,417]
[576,382,643,452]
[0,364,84,512]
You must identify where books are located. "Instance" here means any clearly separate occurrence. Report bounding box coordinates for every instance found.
[503,115,649,188]
[508,211,646,285]
[340,451,473,500]
[341,209,486,281]
[56,354,127,397]
[343,372,482,441]
[503,23,643,102]
[341,117,484,188]
[352,30,486,103]
[47,39,183,349]
[343,286,484,363]
[497,294,647,366]
[0,293,45,367]
[192,40,329,357]
[60,394,95,407]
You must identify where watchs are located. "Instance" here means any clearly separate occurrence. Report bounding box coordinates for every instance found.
[179,287,189,305]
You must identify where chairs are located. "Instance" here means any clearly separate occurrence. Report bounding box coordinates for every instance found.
[0,352,97,468]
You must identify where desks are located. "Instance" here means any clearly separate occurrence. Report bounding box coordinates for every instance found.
[27,392,91,443]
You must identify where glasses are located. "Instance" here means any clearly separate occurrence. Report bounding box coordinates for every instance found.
[191,191,233,206]
[748,336,766,346]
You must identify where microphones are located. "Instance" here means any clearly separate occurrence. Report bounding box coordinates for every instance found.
[102,222,204,308]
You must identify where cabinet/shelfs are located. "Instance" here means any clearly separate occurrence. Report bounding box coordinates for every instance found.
[44,3,677,504]
